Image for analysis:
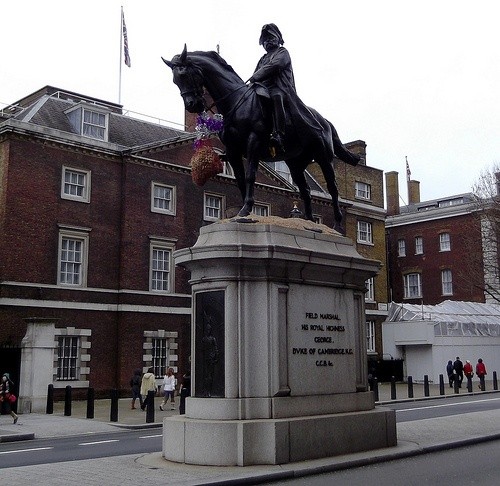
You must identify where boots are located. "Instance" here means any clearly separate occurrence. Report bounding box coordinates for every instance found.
[171,401,176,410]
[160,400,167,411]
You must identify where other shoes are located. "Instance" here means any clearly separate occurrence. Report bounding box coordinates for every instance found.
[14,415,19,424]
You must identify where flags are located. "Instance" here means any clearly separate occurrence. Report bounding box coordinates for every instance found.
[123,13,131,67]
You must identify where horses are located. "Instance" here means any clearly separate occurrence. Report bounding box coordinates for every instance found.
[160,43,362,235]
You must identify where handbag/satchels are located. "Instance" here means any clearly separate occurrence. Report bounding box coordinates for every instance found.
[8,394,17,405]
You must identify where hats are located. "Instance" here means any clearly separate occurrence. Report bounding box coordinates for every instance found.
[3,373,10,379]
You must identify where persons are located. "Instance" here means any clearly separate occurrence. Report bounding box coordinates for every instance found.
[179,370,191,397]
[0,373,18,424]
[129,368,144,409]
[160,367,176,411]
[201,325,219,395]
[476,359,487,388]
[454,357,463,383]
[140,368,157,411]
[447,360,455,388]
[368,368,372,379]
[250,23,327,146]
[463,360,473,388]
